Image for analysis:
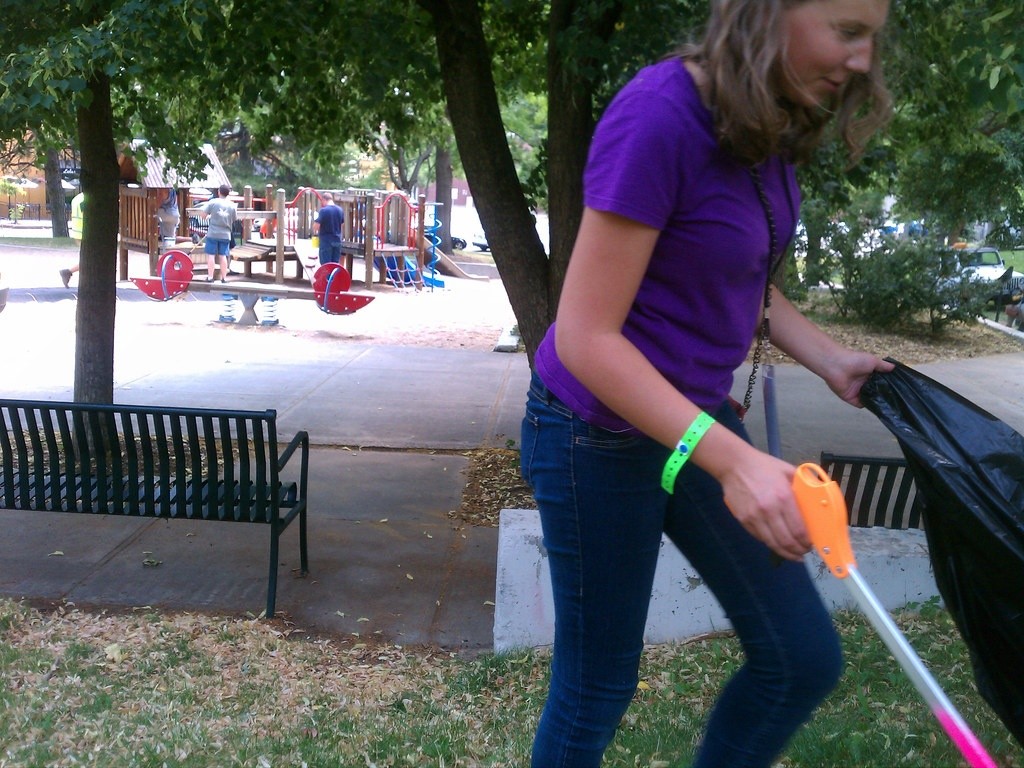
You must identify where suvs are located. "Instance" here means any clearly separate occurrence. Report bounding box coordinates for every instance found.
[932,246,1024,306]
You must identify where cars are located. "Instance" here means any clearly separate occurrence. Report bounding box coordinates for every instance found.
[253,207,321,233]
[472,228,489,253]
[187,202,211,228]
[423,224,467,251]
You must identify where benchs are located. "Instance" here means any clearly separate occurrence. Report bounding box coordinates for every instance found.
[0,398,309,620]
[817,450,921,530]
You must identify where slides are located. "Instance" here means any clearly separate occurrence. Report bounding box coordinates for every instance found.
[372,255,445,288]
[409,227,490,282]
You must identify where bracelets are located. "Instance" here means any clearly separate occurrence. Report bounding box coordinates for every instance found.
[661,412,715,494]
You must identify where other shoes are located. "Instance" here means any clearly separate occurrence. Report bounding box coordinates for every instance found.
[59,269,73,289]
[192,234,203,246]
[203,277,214,283]
[221,279,227,283]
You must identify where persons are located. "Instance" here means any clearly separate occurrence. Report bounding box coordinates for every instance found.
[352,196,366,240]
[260,210,286,239]
[200,185,238,283]
[147,188,180,247]
[60,264,79,289]
[521,0,894,768]
[314,193,344,280]
